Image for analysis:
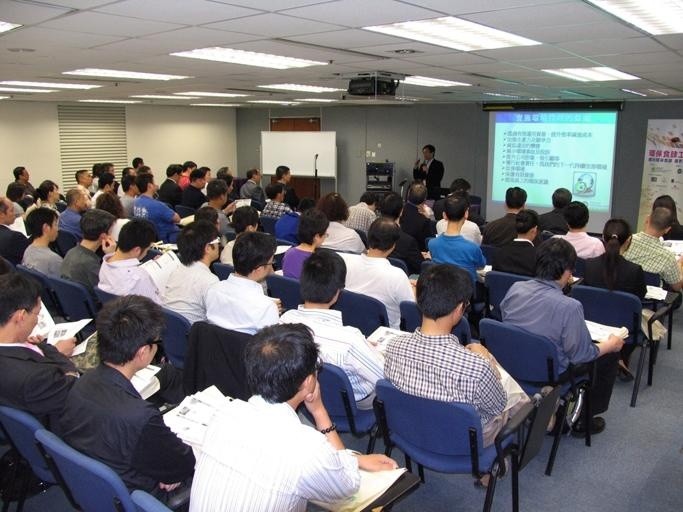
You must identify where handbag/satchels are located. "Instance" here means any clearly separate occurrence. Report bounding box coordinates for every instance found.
[0,447,55,512]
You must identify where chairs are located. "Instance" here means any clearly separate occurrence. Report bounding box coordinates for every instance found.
[0,217,683,512]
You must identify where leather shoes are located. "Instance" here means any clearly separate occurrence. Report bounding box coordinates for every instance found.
[570,415,606,438]
[617,359,634,382]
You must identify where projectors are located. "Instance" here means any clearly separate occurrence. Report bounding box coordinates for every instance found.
[348,78,396,98]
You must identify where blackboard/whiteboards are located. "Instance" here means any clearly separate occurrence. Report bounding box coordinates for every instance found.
[261,130,337,177]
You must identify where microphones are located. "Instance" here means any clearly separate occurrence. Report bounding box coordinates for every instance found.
[314,153,318,160]
[398,177,408,186]
